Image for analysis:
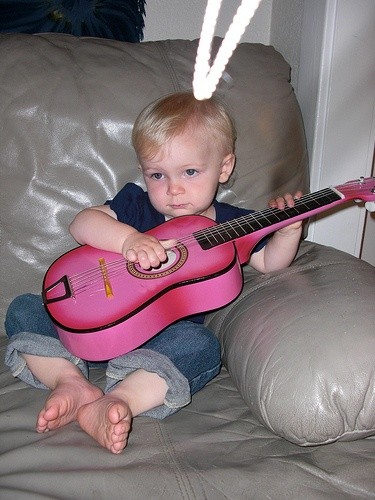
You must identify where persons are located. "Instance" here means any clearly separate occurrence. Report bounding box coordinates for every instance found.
[4,88,304,454]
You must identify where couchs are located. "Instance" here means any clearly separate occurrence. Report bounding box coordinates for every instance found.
[0,34,375,500]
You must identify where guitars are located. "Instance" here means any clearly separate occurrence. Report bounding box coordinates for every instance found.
[42,173,375,362]
